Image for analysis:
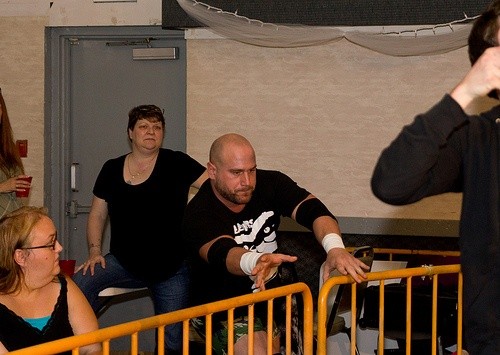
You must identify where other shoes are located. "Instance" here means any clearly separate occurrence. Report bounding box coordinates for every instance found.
[312,310,345,336]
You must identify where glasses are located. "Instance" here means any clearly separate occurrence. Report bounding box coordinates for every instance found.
[17,235,58,250]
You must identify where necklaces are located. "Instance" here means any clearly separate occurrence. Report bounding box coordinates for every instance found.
[127,147,159,184]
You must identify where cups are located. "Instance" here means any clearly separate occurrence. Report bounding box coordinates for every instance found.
[58,260,76,277]
[15,176,31,197]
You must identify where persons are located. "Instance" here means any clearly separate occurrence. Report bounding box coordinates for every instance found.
[182,133,371,355]
[0,91,31,219]
[0,205,102,355]
[370,1,500,355]
[70,105,210,354]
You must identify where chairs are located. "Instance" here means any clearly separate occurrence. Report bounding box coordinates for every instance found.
[313,246,374,355]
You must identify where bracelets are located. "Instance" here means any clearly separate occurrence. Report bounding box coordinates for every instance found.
[87,243,103,249]
